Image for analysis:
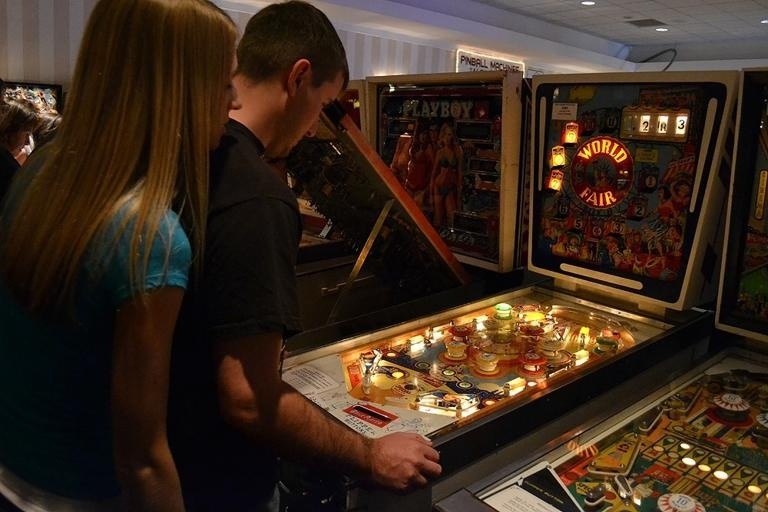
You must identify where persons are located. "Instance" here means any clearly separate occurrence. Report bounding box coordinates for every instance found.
[157,2,444,510]
[605,175,694,271]
[393,117,463,233]
[0,78,65,196]
[1,1,242,512]
